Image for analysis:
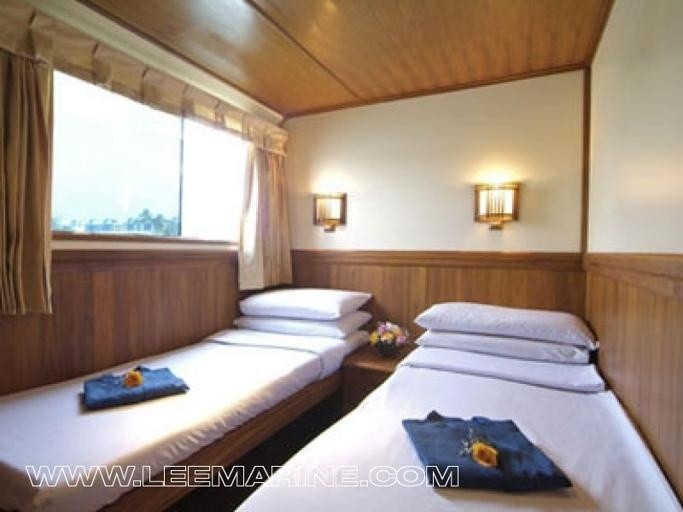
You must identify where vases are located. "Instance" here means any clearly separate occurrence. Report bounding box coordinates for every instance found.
[376,344,402,357]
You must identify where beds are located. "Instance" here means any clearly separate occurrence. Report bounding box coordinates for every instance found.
[234,348,682,510]
[1,328,370,512]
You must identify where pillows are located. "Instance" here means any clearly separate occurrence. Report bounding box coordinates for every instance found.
[232,287,373,339]
[413,301,599,365]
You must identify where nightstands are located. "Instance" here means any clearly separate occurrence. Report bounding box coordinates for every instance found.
[341,347,413,417]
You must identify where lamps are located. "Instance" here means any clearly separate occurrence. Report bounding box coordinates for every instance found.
[473,183,520,230]
[312,193,347,232]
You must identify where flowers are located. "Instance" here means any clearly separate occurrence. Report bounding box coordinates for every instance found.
[367,319,411,347]
